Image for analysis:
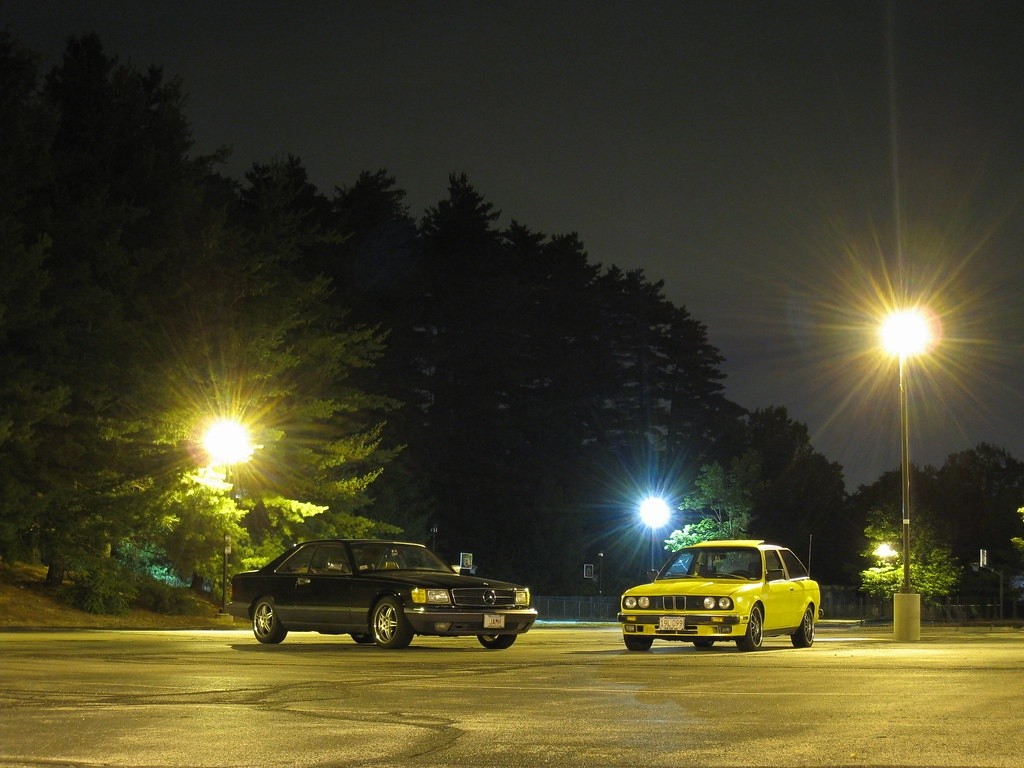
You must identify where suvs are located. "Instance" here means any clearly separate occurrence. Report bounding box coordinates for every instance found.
[616,536,824,654]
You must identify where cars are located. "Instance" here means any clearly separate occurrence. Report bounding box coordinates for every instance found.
[230,537,538,651]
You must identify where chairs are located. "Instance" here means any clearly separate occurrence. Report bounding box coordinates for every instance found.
[382,562,399,569]
[746,562,767,579]
[697,565,718,579]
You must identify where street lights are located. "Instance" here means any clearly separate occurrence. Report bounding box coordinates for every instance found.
[639,497,672,581]
[878,308,934,645]
[201,419,252,622]
